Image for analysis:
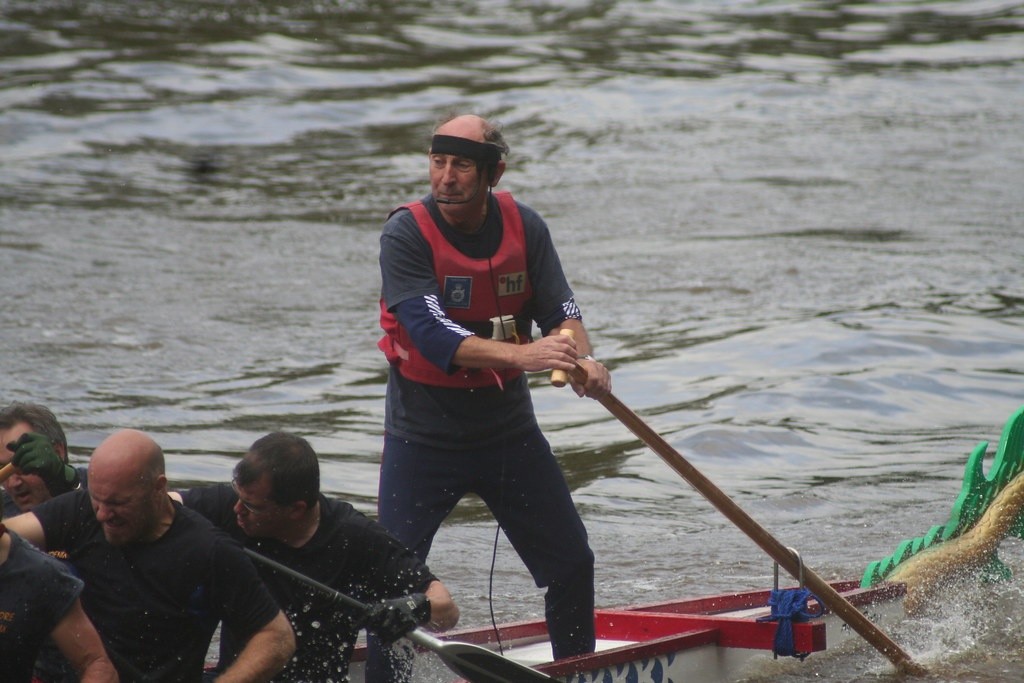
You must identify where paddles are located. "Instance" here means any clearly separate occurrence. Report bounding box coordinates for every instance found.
[105,643,159,682]
[546,327,932,679]
[1,461,22,486]
[243,544,560,683]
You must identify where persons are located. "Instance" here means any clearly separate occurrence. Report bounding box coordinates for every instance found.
[0,520,118,683]
[0,428,297,683]
[0,400,89,522]
[364,115,612,683]
[166,429,461,682]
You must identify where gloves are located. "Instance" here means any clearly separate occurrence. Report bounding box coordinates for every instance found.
[365,593,431,643]
[6,433,80,496]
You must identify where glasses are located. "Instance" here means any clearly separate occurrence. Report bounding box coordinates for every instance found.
[231,477,298,516]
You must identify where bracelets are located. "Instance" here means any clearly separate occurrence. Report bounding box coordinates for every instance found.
[576,353,596,362]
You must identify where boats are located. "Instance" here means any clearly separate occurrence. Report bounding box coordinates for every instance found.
[201,406,1024,683]
